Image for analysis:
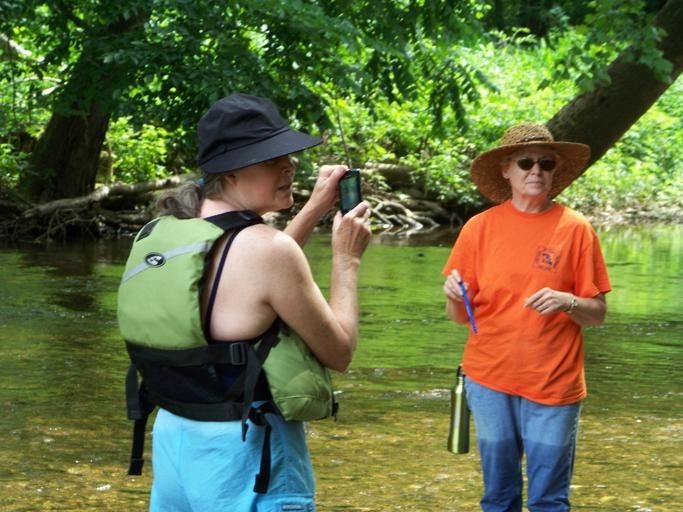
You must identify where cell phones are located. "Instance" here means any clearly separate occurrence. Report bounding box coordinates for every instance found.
[338,169,361,215]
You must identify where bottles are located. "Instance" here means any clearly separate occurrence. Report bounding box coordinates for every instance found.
[446,365,469,455]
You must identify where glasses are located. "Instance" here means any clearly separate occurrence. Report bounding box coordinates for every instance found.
[517,158,557,171]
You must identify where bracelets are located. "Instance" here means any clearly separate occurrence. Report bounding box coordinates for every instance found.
[565,290,578,316]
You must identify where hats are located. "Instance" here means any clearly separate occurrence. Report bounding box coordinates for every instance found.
[470,123,591,205]
[196,92,323,173]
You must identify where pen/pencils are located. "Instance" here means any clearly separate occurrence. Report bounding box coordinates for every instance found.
[459,282,477,333]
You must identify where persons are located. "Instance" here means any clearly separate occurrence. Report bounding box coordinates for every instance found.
[438,119,614,512]
[112,94,373,512]
